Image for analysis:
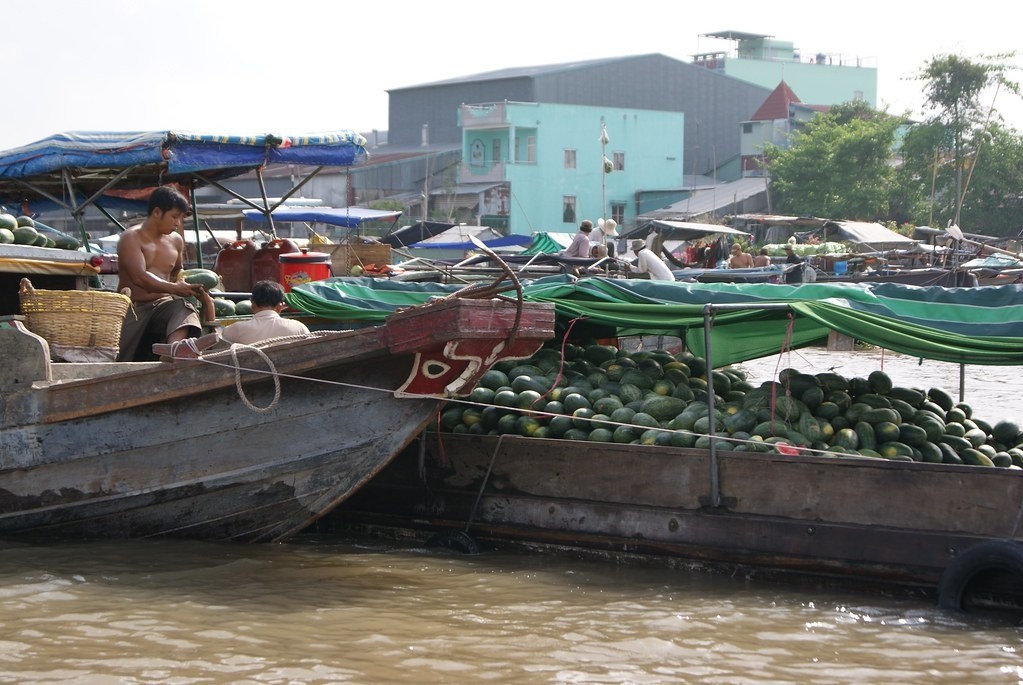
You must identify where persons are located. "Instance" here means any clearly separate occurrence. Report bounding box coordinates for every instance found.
[222,279,309,345]
[754,248,771,267]
[588,218,619,244]
[625,238,675,281]
[731,243,754,268]
[116,186,204,362]
[782,246,802,284]
[589,241,608,258]
[800,258,817,282]
[563,220,592,278]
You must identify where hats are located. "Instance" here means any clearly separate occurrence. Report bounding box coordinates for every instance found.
[581,220,592,229]
[629,239,645,250]
[597,218,619,236]
[597,244,608,259]
[731,243,741,252]
[781,244,797,251]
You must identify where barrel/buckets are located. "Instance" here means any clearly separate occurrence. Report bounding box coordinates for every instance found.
[278,248,335,312]
[832,259,848,276]
[252,238,300,292]
[213,239,256,291]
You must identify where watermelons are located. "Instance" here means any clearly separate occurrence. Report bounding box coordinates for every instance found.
[175,269,255,316]
[423,343,1023,469]
[0,213,81,250]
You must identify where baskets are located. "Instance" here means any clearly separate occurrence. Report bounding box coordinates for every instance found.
[18,278,132,364]
